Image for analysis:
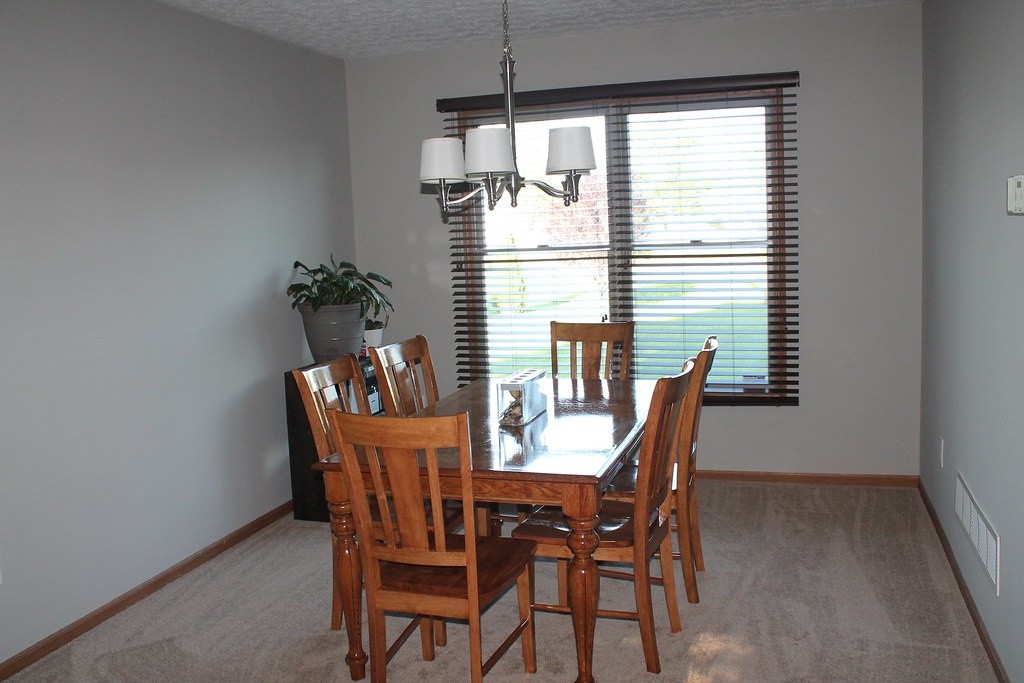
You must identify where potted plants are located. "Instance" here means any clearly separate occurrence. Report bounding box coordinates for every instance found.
[287,252,394,364]
[364,317,385,356]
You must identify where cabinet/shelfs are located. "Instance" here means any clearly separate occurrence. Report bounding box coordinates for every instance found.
[284,364,383,522]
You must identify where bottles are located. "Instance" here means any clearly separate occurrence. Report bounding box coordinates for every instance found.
[359,340,367,359]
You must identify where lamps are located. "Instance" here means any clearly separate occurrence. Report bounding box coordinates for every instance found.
[419,1,597,224]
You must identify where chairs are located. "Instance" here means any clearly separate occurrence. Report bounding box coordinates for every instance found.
[511,360,695,674]
[292,352,464,647]
[325,410,537,683]
[368,334,491,538]
[517,321,634,526]
[601,334,718,604]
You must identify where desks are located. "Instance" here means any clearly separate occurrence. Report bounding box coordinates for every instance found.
[309,377,658,683]
